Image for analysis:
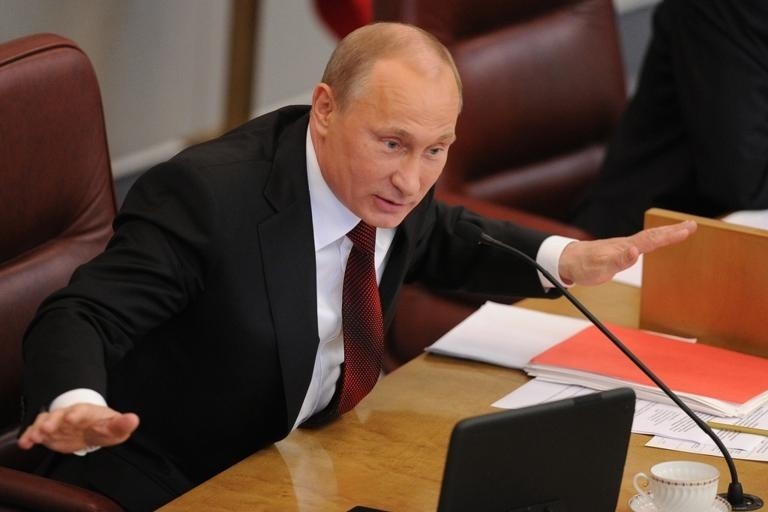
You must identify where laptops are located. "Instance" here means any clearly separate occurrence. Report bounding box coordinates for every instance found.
[436,388,635,512]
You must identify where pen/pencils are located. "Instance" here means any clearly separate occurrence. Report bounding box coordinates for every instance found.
[706,420,767,438]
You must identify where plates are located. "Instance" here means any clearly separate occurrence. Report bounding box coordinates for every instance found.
[628,491,732,512]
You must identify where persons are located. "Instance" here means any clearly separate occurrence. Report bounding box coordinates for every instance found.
[541,1,768,241]
[14,21,698,512]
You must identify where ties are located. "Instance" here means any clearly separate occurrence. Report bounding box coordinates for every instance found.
[295,221,385,430]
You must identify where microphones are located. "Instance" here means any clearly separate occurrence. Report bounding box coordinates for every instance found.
[454,220,763,510]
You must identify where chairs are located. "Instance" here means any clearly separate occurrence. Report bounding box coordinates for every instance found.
[381,1,626,380]
[3,27,180,512]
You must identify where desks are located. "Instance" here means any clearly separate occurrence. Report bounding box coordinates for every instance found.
[155,208,768,512]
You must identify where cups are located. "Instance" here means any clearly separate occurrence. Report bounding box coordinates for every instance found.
[633,461,720,512]
